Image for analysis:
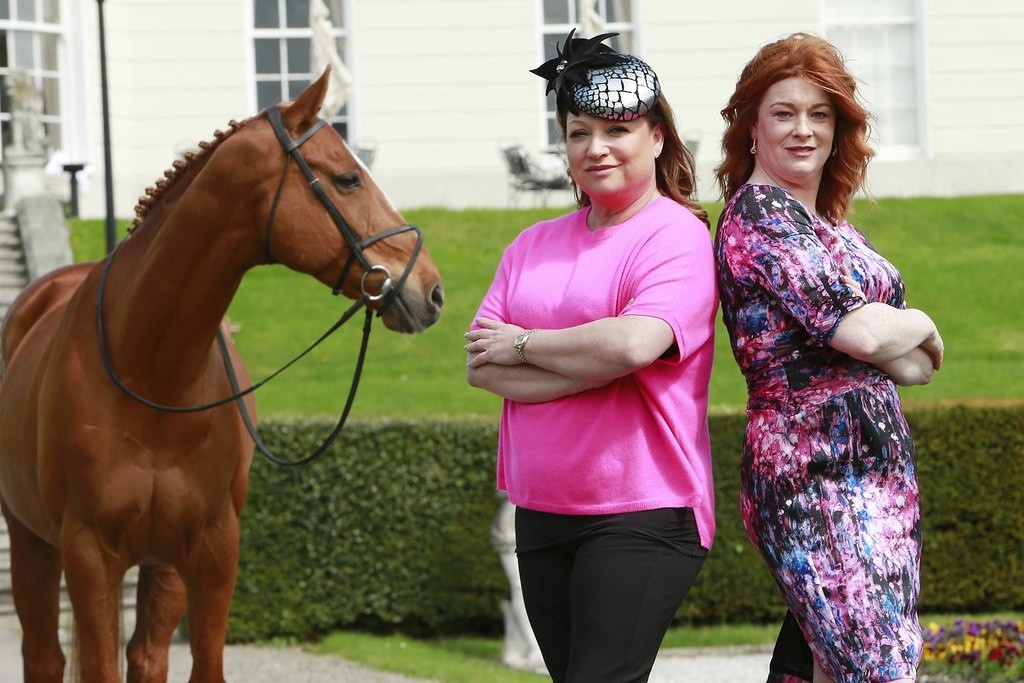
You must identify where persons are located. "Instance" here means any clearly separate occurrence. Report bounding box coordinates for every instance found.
[462,29,719,682]
[711,30,944,683]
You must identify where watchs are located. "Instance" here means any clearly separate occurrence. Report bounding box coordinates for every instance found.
[514,329,541,363]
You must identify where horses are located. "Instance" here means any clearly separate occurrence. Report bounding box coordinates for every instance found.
[0,61,444,683]
